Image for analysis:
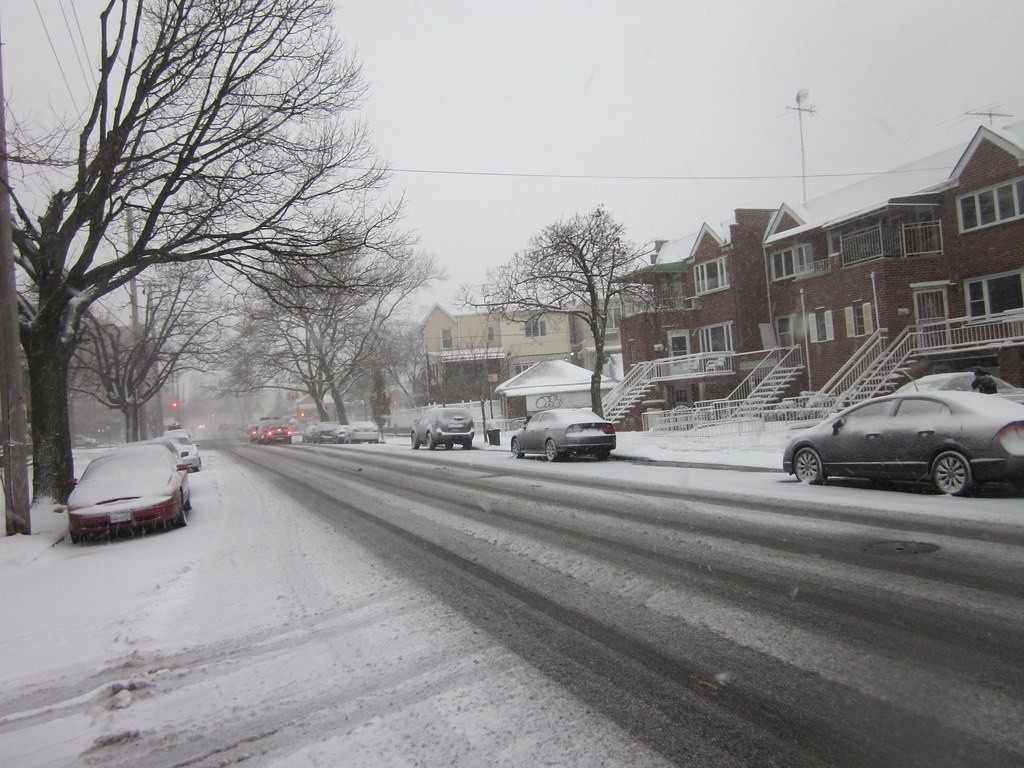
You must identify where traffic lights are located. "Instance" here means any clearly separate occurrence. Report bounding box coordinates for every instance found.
[301,412,305,417]
[172,402,178,408]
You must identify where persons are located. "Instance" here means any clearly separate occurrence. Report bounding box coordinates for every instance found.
[971,366,998,394]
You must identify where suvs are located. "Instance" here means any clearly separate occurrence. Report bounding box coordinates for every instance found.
[410,407,476,451]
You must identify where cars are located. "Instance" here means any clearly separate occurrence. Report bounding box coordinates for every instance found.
[65,448,193,543]
[510,408,617,462]
[783,388,1024,499]
[890,372,1024,408]
[247,417,292,445]
[302,421,350,445]
[72,435,98,448]
[344,421,379,445]
[124,420,202,474]
